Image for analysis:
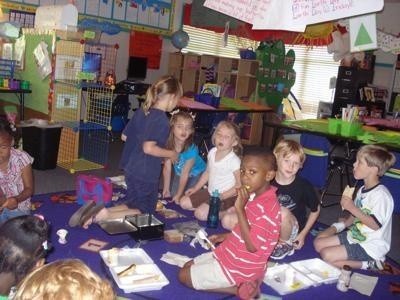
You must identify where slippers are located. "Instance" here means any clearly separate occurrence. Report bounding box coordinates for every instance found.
[80,204,106,229]
[69,199,96,228]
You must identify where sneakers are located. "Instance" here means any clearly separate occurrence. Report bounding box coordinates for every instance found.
[235,281,260,300]
[269,242,295,260]
[365,259,384,271]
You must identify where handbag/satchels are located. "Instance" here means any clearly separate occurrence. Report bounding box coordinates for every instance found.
[194,88,221,108]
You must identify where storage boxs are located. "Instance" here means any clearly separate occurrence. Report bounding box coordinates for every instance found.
[298,134,331,188]
[381,153,400,213]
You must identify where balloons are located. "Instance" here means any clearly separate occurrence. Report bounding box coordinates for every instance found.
[172,30,189,49]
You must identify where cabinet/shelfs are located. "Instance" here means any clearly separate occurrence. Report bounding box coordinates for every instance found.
[181,54,198,92]
[332,67,374,118]
[168,54,181,72]
[201,56,216,84]
[235,59,259,97]
[217,57,233,85]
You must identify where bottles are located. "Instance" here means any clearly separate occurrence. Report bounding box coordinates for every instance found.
[207,189,220,228]
[336,265,352,293]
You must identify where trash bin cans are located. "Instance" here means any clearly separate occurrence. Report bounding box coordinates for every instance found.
[20,119,62,170]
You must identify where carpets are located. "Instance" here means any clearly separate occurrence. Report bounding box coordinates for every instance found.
[32,190,400,300]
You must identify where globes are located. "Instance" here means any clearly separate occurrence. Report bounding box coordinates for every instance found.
[172,31,189,54]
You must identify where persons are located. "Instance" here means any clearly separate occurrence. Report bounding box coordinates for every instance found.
[0,214,53,300]
[179,120,241,221]
[269,139,321,260]
[12,258,116,300]
[0,106,34,223]
[177,148,282,300]
[68,75,183,230]
[313,145,394,271]
[162,109,205,205]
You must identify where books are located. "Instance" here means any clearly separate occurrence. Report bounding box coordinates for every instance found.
[341,104,368,124]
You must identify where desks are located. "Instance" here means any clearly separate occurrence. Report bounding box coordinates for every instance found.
[266,117,400,208]
[0,85,32,121]
[181,96,274,156]
[82,80,150,142]
